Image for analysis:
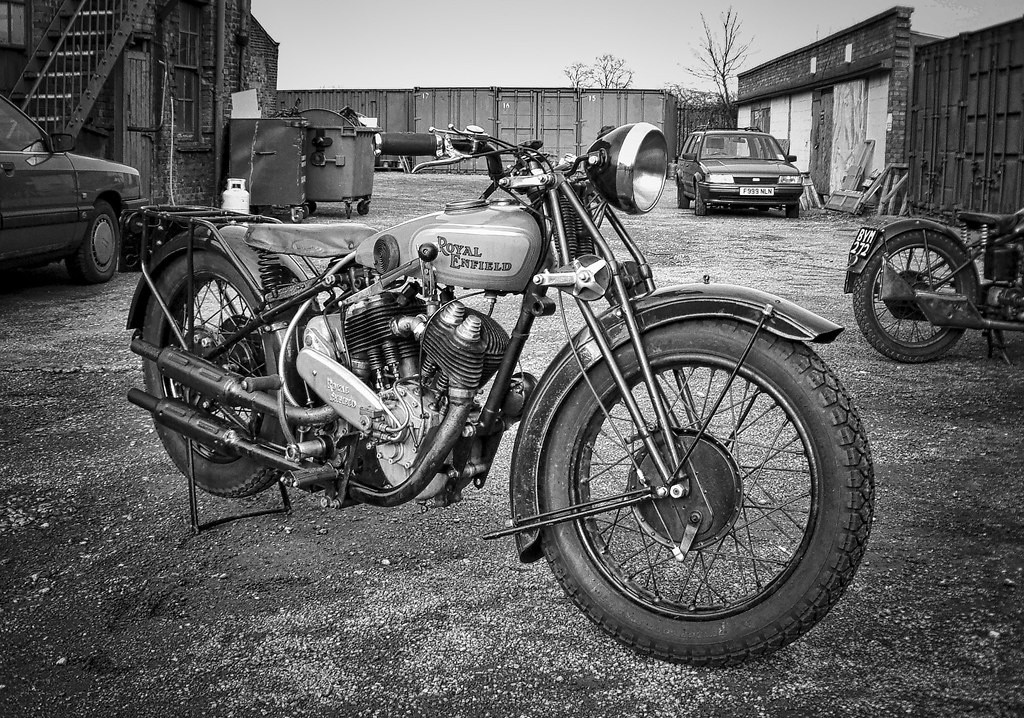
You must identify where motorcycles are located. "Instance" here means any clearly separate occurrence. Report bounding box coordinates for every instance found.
[128,120,875,667]
[847,201,1023,362]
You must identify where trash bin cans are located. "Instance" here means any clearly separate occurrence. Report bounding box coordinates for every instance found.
[227,116,313,222]
[293,106,384,219]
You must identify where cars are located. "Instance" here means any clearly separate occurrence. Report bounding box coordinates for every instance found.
[0,92,140,283]
[673,126,806,219]
